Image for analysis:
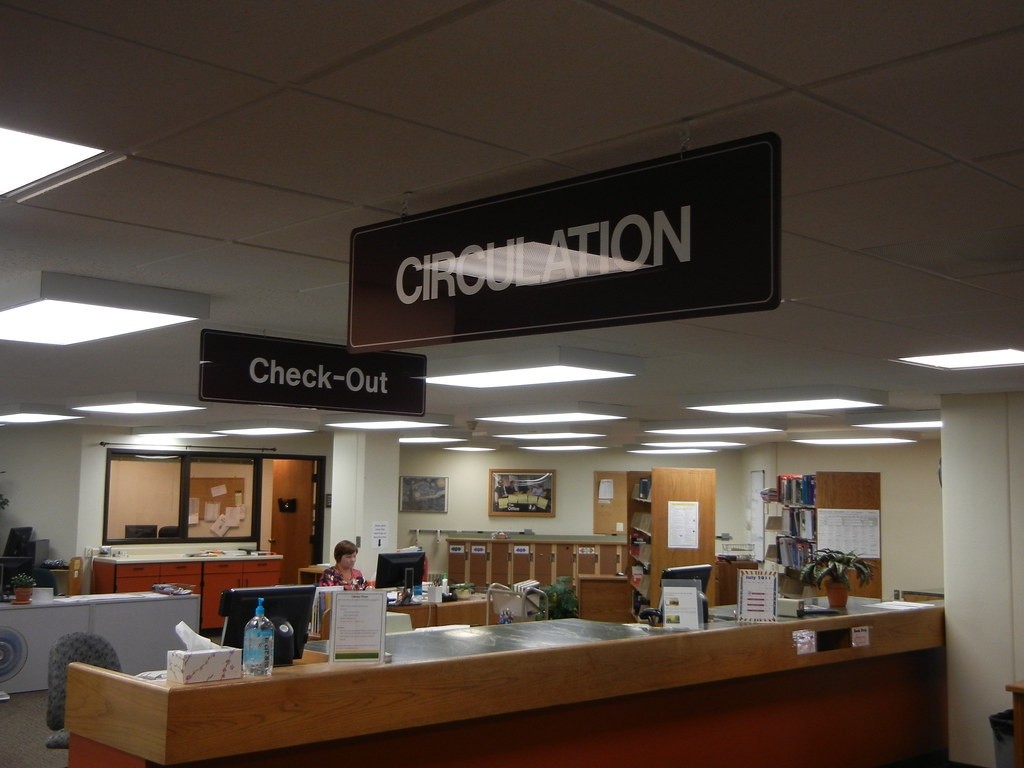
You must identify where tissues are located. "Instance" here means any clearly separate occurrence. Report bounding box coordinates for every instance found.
[167,621,242,684]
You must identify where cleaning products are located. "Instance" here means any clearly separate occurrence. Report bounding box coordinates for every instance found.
[242,598,275,676]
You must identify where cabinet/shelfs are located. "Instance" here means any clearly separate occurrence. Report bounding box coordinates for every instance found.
[593,467,716,607]
[765,472,882,600]
[93,554,284,629]
[447,534,627,591]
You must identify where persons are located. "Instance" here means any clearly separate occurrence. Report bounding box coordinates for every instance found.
[317,540,367,591]
[495,480,543,512]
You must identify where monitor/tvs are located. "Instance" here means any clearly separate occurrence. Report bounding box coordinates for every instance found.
[375,552,425,606]
[124,525,157,538]
[220,585,317,668]
[657,563,711,623]
[3,527,32,556]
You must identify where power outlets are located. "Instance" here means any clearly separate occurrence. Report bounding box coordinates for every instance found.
[85,547,92,557]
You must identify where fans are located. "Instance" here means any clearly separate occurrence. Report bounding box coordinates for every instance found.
[0,626,29,702]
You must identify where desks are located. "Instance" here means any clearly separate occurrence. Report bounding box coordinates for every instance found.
[383,589,501,626]
[0,589,200,694]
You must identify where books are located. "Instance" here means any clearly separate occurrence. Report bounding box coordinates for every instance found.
[761,474,815,569]
[627,476,653,617]
[311,585,344,634]
[514,579,540,598]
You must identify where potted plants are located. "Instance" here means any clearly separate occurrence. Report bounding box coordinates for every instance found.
[10,573,33,604]
[800,546,879,609]
[453,583,473,598]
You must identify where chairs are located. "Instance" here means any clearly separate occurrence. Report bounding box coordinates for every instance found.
[44,632,121,749]
[157,525,181,539]
[32,568,58,596]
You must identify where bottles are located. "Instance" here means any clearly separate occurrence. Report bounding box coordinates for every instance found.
[442,573,448,585]
[414,586,422,595]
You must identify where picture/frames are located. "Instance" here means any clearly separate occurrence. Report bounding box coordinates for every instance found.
[488,469,556,517]
